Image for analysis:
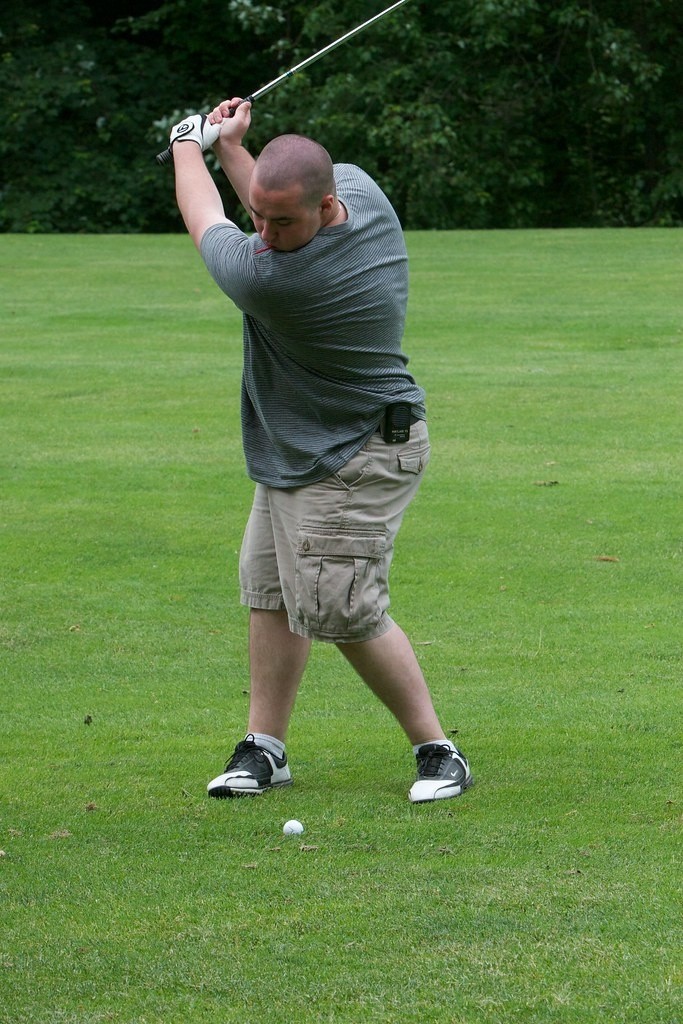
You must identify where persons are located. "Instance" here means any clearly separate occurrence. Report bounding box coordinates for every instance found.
[170,96,471,803]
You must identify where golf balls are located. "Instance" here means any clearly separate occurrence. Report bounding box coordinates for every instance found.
[282,819,304,836]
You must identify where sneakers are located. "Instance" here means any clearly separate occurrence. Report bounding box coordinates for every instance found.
[207,733,293,800]
[408,744,473,803]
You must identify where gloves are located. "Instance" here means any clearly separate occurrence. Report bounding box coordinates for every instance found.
[170,114,230,152]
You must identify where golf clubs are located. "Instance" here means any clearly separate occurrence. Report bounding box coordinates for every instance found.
[154,1,408,168]
